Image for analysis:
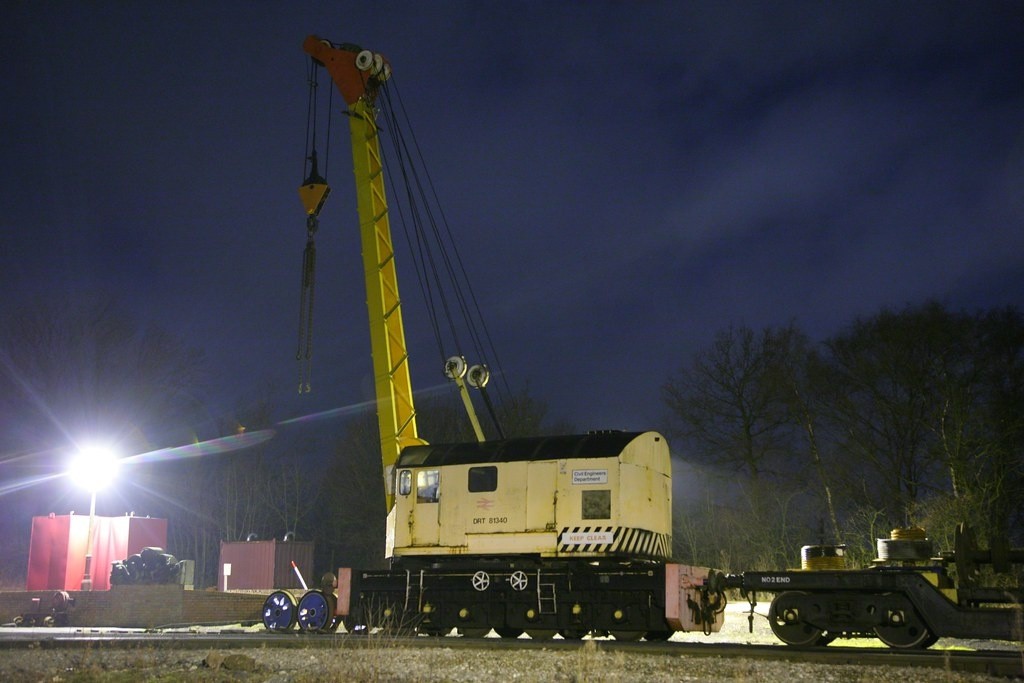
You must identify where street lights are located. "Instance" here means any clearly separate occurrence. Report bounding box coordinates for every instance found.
[66,441,118,590]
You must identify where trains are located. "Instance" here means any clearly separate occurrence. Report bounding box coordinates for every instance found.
[339,429,1024,650]
